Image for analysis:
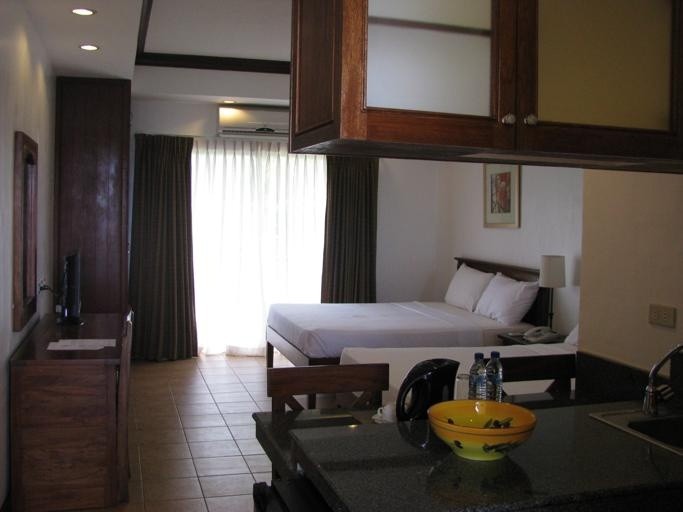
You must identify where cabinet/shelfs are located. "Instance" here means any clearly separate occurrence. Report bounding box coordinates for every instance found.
[288,1,682,178]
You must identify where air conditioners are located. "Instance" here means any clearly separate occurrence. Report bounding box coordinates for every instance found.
[216,105,289,141]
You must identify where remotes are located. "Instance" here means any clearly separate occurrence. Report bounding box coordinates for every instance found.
[508,331,526,337]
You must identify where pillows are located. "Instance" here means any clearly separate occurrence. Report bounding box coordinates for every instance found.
[442,263,494,313]
[473,271,541,327]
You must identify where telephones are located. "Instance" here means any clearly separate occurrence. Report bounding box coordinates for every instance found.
[522,327,564,344]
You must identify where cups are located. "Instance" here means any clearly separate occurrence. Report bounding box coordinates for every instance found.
[454,371,470,401]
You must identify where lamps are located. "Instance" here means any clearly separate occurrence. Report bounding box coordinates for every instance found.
[538,254,565,327]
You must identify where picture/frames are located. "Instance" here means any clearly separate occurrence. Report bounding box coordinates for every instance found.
[482,162,521,228]
[14,130,38,332]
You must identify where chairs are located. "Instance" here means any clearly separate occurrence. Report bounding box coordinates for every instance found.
[115,308,136,503]
[471,348,577,411]
[266,360,387,412]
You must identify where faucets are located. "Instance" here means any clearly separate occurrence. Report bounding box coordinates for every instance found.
[642,343,683,416]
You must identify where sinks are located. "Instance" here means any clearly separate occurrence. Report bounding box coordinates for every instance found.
[588,408,683,456]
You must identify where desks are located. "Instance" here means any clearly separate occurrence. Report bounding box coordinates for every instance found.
[242,397,683,510]
[6,311,125,510]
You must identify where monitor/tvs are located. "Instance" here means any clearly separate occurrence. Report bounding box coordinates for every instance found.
[63,252,80,335]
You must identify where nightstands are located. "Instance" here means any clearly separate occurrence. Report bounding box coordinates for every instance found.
[497,330,569,345]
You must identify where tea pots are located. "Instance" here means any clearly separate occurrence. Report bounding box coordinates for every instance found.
[378,400,408,421]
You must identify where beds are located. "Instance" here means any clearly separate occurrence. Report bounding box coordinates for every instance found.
[265,256,551,410]
[335,318,579,412]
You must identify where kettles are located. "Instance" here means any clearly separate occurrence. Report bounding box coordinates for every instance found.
[396,356,460,441]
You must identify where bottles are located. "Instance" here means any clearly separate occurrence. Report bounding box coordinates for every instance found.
[467,349,502,402]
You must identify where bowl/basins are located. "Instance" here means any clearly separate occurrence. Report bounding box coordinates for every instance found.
[427,399,540,462]
[423,449,534,503]
